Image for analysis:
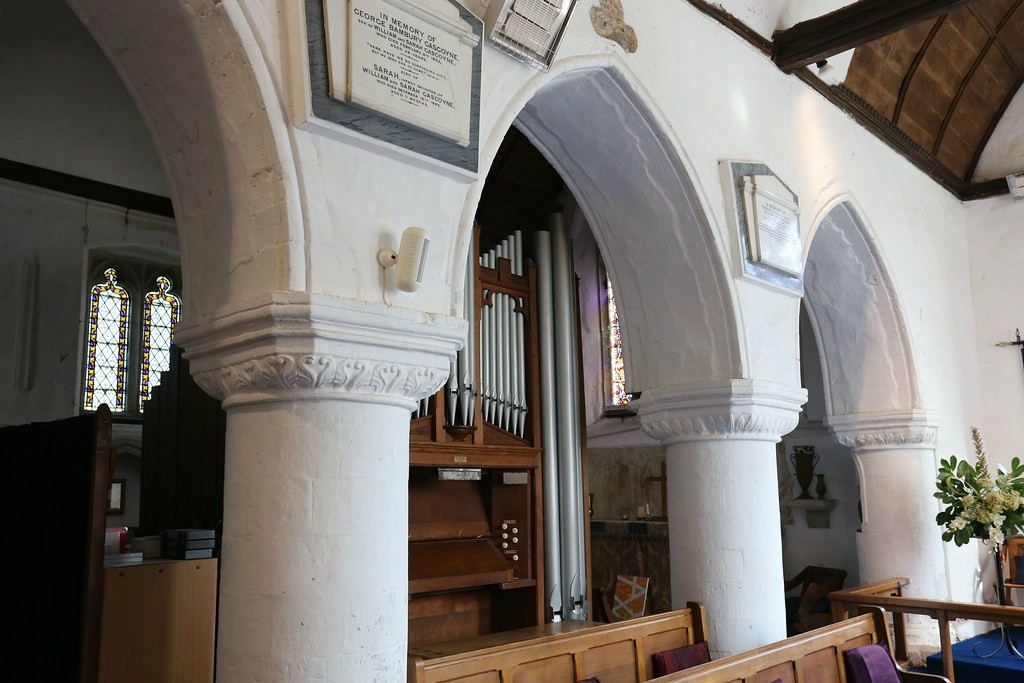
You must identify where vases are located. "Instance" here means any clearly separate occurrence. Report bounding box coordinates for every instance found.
[970,519,986,537]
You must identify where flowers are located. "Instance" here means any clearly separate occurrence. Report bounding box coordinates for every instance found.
[931,422,1024,551]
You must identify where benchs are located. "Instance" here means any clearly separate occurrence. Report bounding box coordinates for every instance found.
[636,603,954,683]
[408,597,714,683]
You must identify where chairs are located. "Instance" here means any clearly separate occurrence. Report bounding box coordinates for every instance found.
[785,564,849,631]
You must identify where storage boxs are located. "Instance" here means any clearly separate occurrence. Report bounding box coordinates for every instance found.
[130,528,218,560]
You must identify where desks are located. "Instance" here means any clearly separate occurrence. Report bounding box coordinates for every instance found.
[925,626,1024,683]
[92,555,218,683]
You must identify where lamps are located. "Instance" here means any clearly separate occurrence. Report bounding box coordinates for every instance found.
[372,221,437,307]
